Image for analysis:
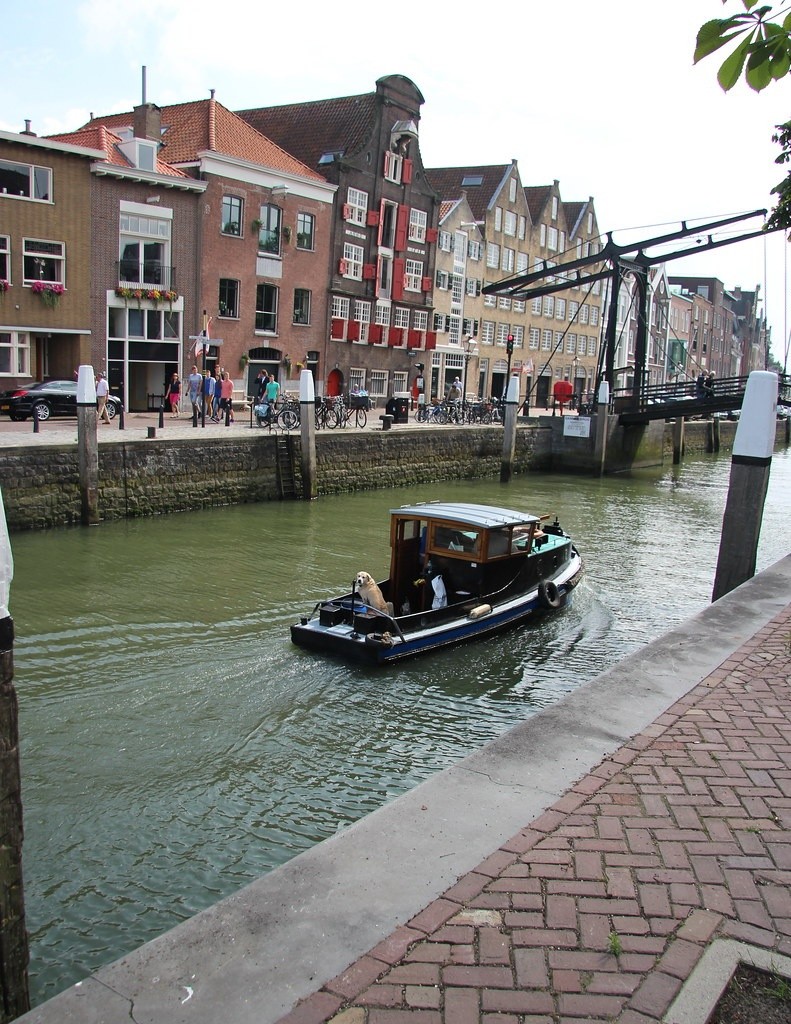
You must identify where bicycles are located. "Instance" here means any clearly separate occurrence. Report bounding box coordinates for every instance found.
[254,392,368,430]
[414,395,504,425]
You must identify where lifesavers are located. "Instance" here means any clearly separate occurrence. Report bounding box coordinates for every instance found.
[538,580,561,610]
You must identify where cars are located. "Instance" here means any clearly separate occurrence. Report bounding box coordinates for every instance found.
[645,395,791,420]
[0,378,123,421]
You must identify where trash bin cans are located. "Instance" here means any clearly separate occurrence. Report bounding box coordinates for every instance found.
[386,397,409,423]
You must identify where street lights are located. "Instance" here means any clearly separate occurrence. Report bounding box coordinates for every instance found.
[461,335,477,417]
[572,356,581,405]
[674,365,682,392]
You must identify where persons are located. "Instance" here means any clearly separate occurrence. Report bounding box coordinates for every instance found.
[452,377,462,397]
[211,372,234,423]
[262,375,280,408]
[419,527,473,565]
[255,370,269,401]
[165,373,182,418]
[359,385,374,410]
[474,532,518,555]
[696,369,708,397]
[705,371,716,397]
[446,385,461,401]
[186,365,203,419]
[198,366,224,419]
[351,384,359,397]
[96,373,110,424]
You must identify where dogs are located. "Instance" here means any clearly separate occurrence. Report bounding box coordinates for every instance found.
[356,572,389,617]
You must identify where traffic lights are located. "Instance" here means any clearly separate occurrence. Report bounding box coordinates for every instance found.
[506,334,514,354]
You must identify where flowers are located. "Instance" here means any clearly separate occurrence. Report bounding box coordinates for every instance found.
[31,282,63,311]
[0,280,10,297]
[115,287,178,320]
[254,219,263,226]
[284,226,292,243]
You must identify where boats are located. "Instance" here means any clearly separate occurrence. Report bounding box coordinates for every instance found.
[290,499,586,666]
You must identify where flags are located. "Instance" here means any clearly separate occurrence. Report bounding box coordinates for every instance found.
[195,325,209,357]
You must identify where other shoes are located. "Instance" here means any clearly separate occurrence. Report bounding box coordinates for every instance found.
[102,422,110,424]
[198,411,201,419]
[189,416,193,419]
[170,415,176,418]
[211,417,220,423]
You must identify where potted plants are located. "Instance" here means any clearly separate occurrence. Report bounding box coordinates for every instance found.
[239,353,250,372]
